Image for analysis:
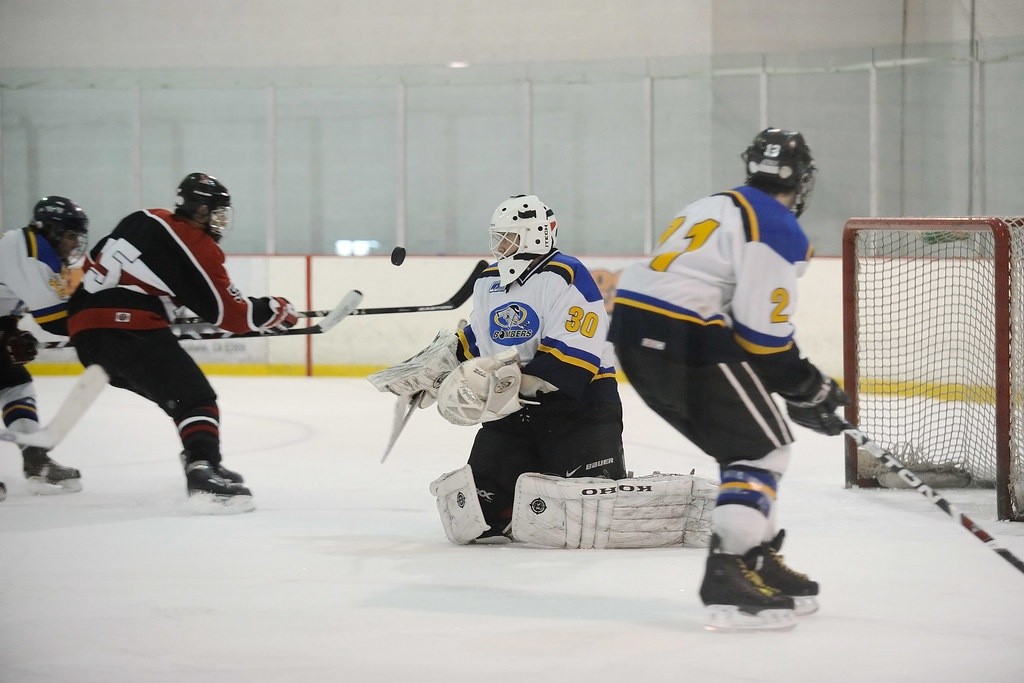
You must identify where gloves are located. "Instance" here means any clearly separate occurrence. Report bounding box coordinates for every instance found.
[778,363,852,436]
[258,297,299,336]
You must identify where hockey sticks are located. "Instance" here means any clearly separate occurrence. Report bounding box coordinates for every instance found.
[1,362,109,451]
[379,389,426,465]
[168,260,493,325]
[834,415,1024,574]
[36,289,364,350]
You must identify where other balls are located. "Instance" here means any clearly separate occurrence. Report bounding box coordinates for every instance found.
[390,246,406,266]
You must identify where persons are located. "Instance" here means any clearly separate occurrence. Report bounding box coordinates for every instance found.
[369,196,626,544]
[612,129,852,630]
[67,174,298,516]
[0,195,88,499]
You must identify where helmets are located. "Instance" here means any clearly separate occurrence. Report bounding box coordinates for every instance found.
[489,194,559,286]
[32,197,89,265]
[176,173,232,236]
[743,127,815,218]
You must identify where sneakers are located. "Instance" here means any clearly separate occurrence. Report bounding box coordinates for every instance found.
[743,527,819,616]
[697,538,795,632]
[179,451,254,514]
[21,448,83,497]
